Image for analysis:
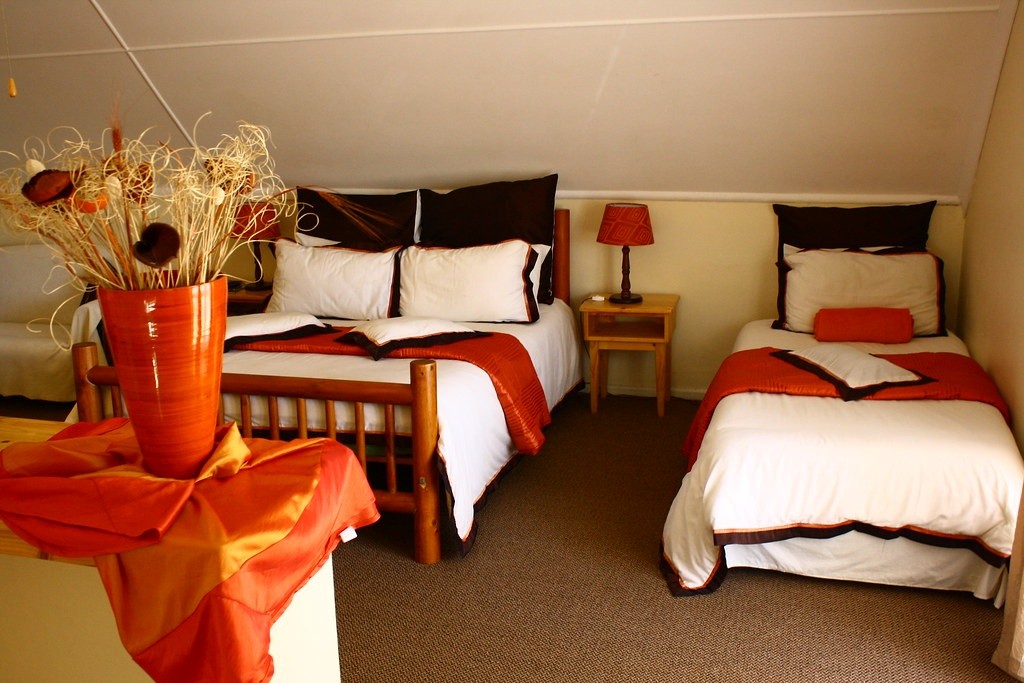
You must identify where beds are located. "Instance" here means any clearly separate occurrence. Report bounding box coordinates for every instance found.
[70,207,586,568]
[657,318,1024,609]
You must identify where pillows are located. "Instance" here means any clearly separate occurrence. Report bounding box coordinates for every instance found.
[393,236,541,325]
[780,243,949,339]
[813,306,918,345]
[264,235,404,322]
[770,199,939,337]
[413,172,560,308]
[292,181,419,319]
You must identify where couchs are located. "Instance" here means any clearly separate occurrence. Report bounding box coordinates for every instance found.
[0,199,126,402]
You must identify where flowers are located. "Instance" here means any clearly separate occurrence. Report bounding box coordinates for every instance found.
[0,107,401,291]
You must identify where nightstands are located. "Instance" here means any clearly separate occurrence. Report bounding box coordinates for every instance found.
[579,290,680,417]
[227,291,272,314]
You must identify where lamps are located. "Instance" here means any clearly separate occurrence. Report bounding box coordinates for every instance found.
[226,201,284,293]
[595,202,655,305]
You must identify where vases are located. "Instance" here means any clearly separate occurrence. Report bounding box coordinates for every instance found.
[91,266,230,482]
